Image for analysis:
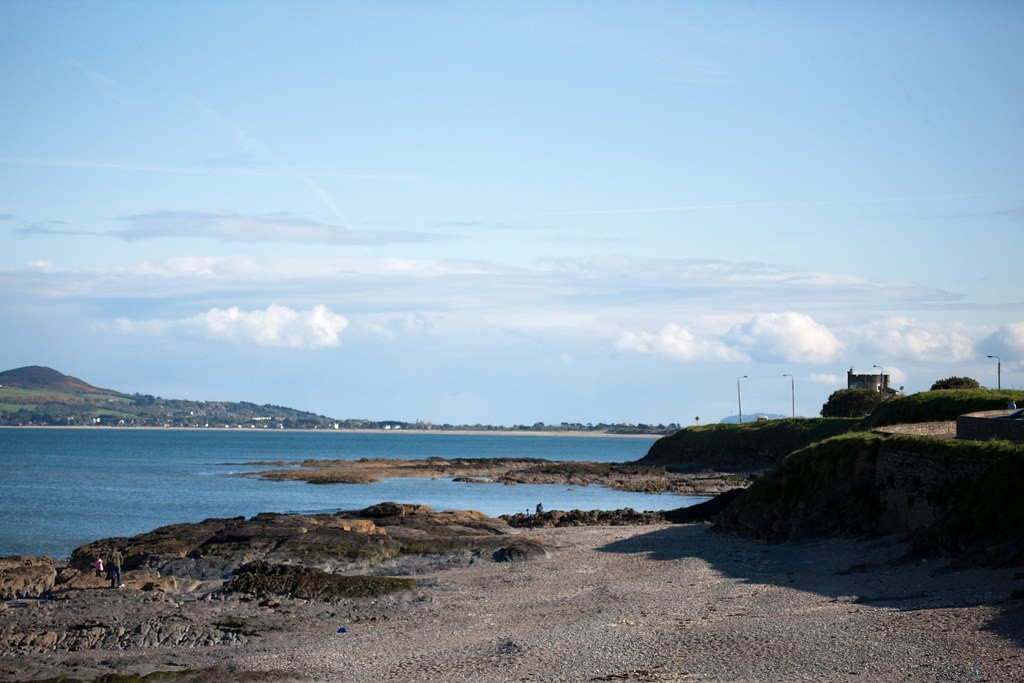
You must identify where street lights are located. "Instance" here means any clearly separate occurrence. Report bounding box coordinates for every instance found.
[873,365,884,402]
[783,374,794,418]
[737,375,748,424]
[987,355,1001,391]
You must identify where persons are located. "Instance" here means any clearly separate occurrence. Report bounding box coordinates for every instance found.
[1007,400,1017,410]
